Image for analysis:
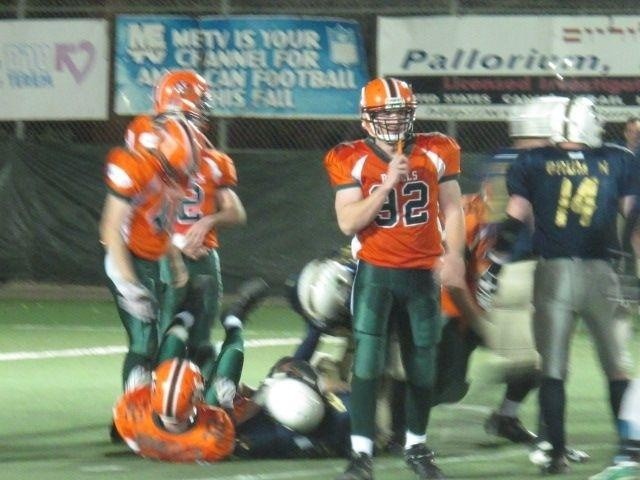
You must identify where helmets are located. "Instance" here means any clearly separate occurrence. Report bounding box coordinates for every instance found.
[553,96,603,148]
[266,376,325,432]
[151,358,205,422]
[124,71,214,176]
[298,260,355,329]
[359,77,417,147]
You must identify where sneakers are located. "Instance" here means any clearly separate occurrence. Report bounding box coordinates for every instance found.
[337,451,372,478]
[527,441,569,473]
[221,276,268,326]
[484,413,539,444]
[403,442,443,478]
